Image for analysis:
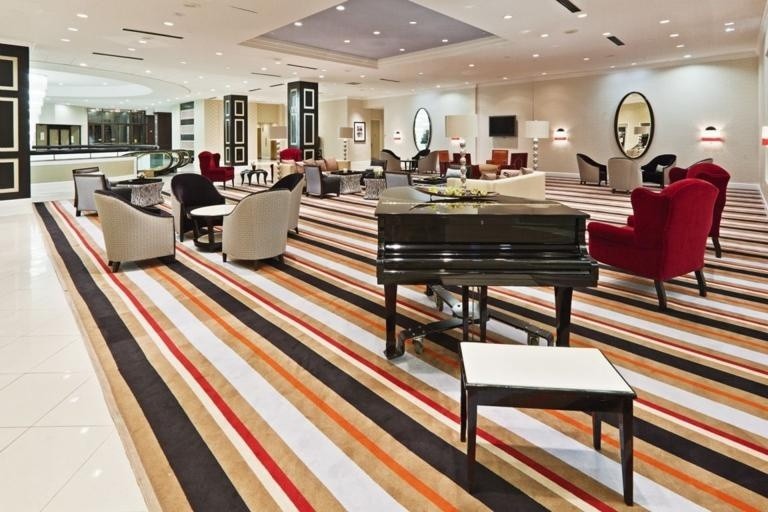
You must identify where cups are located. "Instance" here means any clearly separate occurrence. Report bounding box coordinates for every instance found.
[342,168,348,174]
[252,165,256,171]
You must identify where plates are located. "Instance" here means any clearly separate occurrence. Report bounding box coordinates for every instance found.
[408,198,498,213]
[412,184,499,200]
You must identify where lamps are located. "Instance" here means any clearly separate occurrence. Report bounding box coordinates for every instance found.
[699,123,724,144]
[443,113,479,189]
[525,120,549,171]
[338,126,353,160]
[552,126,567,141]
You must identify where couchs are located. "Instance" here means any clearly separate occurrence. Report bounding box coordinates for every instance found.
[574,152,730,313]
[71,148,304,274]
[279,148,545,202]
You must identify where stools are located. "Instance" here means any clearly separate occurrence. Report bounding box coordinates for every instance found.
[456,341,637,507]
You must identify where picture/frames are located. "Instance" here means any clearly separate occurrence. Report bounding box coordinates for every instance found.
[354,121,366,142]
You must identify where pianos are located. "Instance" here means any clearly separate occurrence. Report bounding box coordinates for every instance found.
[376,186,599,358]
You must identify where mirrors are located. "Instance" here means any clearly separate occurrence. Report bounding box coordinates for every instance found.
[614,90,655,159]
[411,107,432,152]
